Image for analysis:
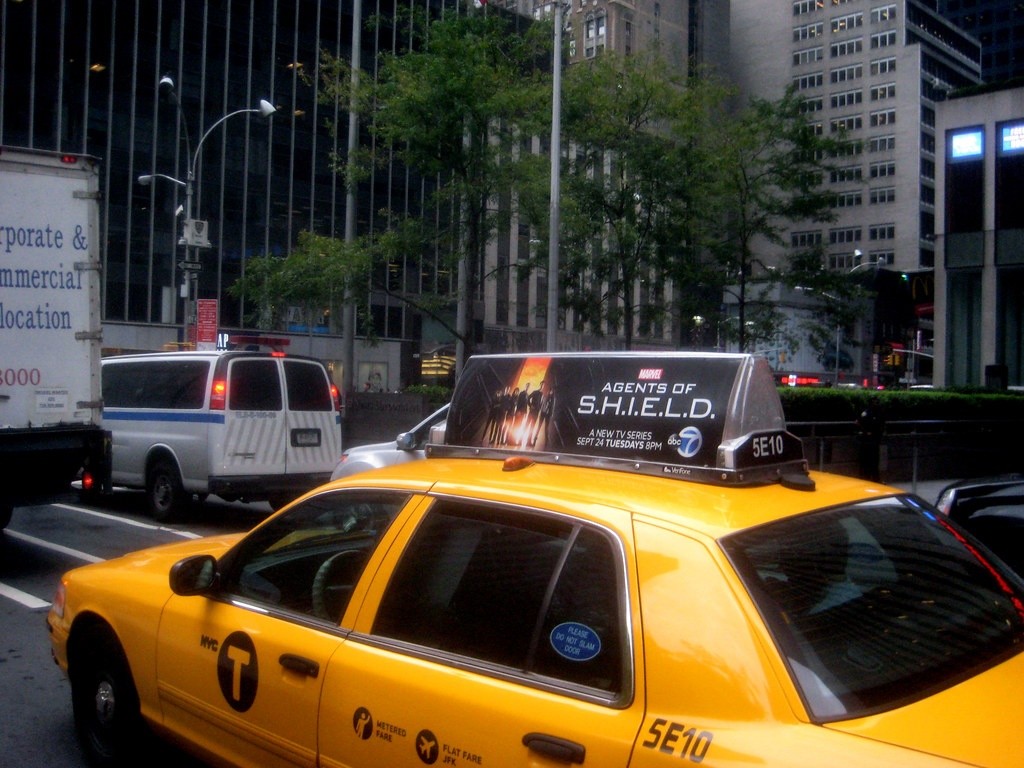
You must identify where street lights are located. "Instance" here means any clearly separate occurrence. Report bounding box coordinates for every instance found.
[717,317,740,352]
[138,75,277,342]
[794,249,886,388]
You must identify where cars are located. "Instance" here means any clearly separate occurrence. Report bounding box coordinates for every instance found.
[47,351,1024,768]
[935,466,1024,580]
[328,401,450,483]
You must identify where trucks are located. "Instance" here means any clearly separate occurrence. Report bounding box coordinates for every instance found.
[1,144,103,530]
[102,351,343,524]
